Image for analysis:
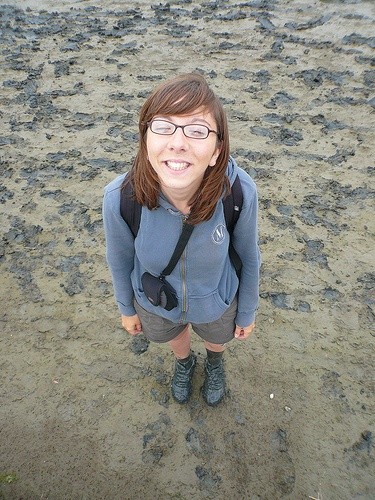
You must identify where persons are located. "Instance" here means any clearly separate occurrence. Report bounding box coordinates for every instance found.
[102,73,262,408]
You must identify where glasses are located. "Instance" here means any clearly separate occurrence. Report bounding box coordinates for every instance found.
[146,117,221,140]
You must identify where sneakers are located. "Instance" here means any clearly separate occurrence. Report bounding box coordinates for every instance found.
[202,356,227,406]
[170,351,197,403]
